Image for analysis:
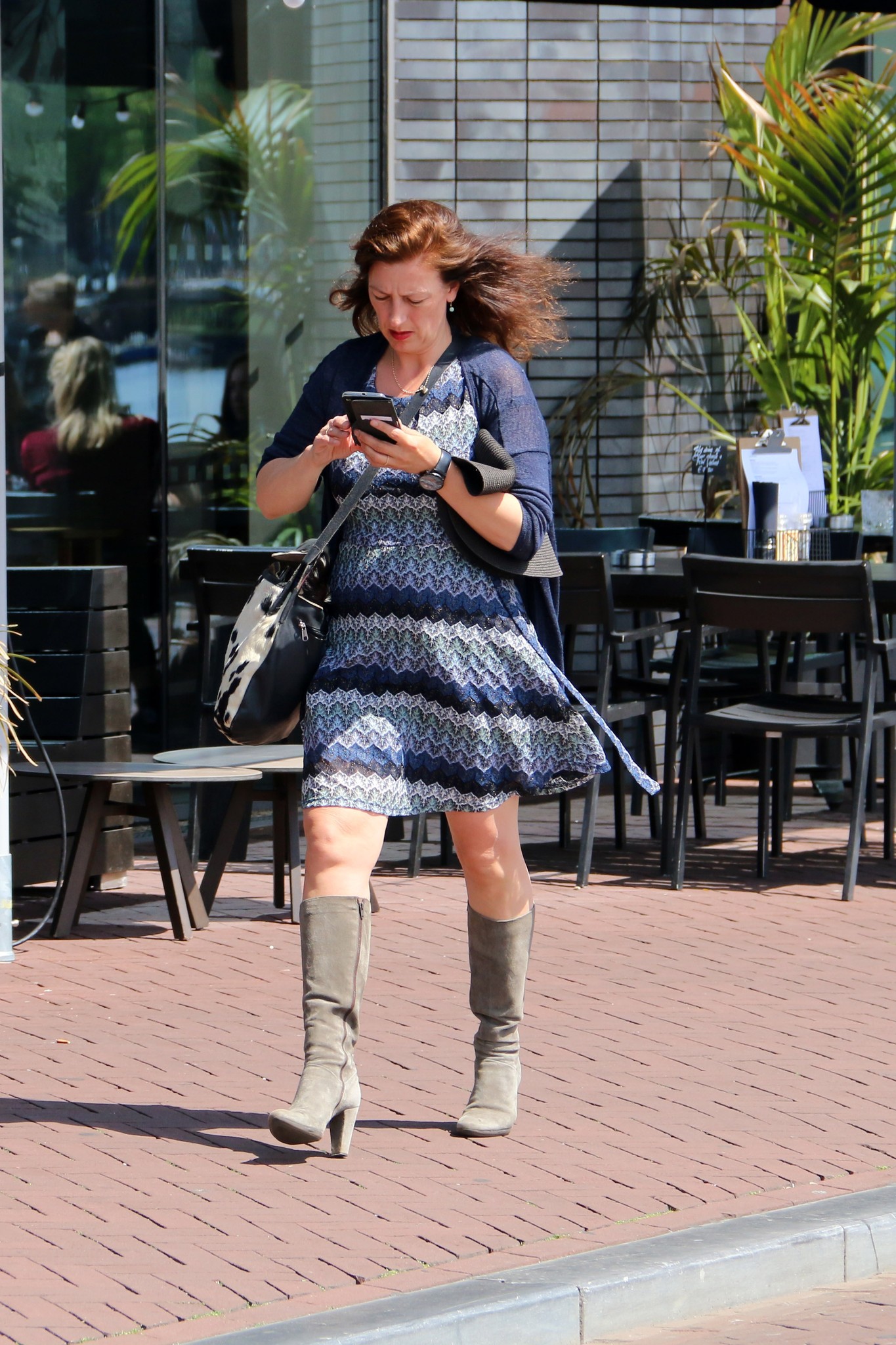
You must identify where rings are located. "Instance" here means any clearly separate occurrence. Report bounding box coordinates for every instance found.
[383,455,389,466]
[325,427,331,435]
[331,418,334,426]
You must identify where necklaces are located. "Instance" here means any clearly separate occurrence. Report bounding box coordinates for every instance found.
[391,348,434,394]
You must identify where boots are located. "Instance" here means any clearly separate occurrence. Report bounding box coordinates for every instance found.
[455,901,535,1136]
[266,895,371,1156]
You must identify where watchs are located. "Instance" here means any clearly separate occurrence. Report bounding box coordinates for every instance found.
[418,448,452,491]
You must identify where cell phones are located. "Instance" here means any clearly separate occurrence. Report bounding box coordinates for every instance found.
[341,391,402,448]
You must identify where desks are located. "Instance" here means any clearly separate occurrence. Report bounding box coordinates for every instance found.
[9,760,262,938]
[605,556,896,845]
[153,745,378,922]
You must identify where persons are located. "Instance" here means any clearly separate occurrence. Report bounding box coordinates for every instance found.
[21,336,162,755]
[4,271,96,475]
[256,200,661,1156]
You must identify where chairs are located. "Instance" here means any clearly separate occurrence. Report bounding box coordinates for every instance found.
[9,512,895,941]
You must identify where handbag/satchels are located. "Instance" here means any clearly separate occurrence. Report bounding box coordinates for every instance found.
[209,561,332,749]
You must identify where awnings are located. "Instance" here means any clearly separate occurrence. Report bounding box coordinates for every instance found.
[1,0,249,90]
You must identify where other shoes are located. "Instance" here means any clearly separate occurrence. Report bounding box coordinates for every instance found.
[131,670,165,734]
